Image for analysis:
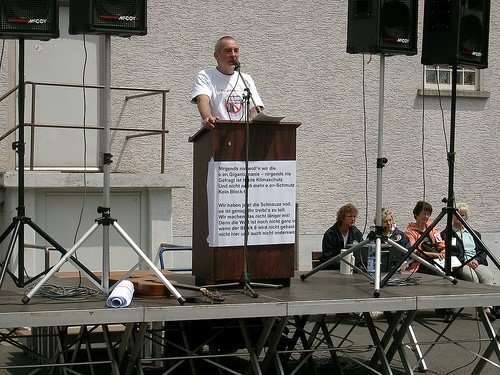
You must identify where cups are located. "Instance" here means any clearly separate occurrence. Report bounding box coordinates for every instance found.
[339,248,355,275]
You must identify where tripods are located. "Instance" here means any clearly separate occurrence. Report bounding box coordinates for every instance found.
[0,34,187,306]
[200,67,284,300]
[300,53,500,298]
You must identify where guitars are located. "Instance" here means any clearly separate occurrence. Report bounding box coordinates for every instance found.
[108,269,226,304]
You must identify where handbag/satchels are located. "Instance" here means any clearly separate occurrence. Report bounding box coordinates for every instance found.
[421,242,439,253]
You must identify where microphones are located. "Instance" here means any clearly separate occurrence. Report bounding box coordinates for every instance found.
[233,59,241,67]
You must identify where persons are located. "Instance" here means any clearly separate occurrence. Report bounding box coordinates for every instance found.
[190,36,265,130]
[322,201,500,324]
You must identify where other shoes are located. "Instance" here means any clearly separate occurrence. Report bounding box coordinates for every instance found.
[358,320,368,327]
[444,312,454,323]
[399,318,408,324]
[337,317,345,324]
[478,309,496,321]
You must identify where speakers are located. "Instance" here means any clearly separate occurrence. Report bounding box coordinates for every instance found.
[345,0,419,57]
[68,0,148,38]
[420,0,491,69]
[0,0,60,42]
